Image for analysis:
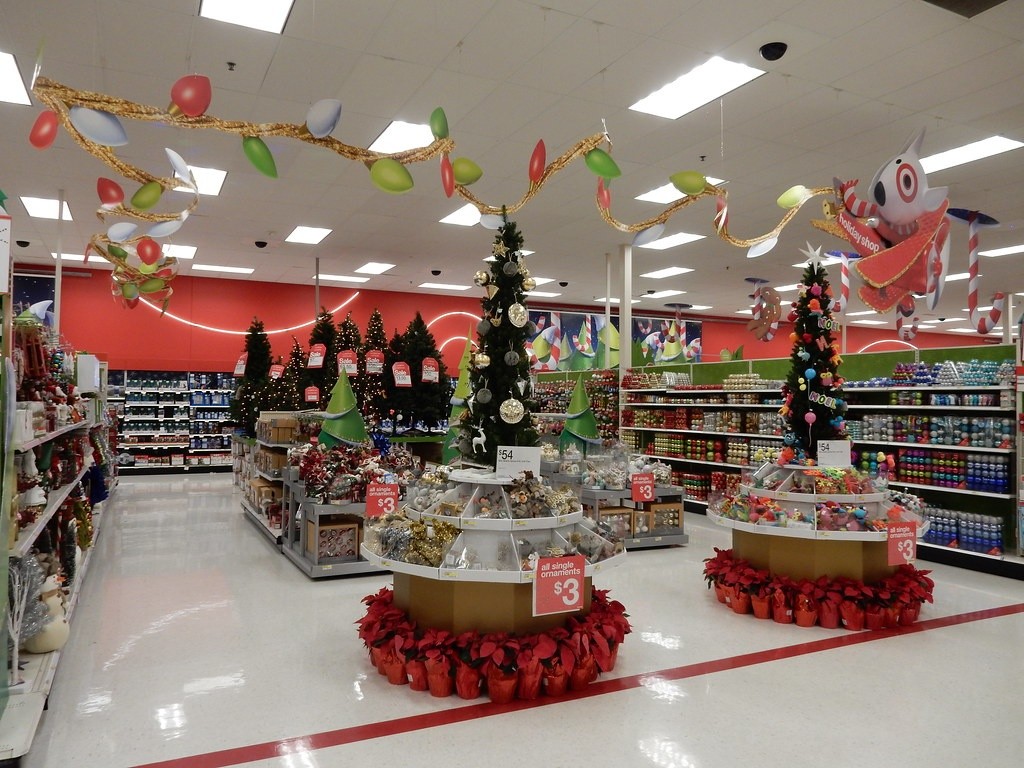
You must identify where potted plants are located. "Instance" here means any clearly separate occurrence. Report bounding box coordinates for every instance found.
[354,582,633,703]
[702,546,934,632]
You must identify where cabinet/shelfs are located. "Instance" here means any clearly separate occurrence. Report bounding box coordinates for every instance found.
[282,466,409,580]
[724,389,785,494]
[230,433,295,555]
[0,416,118,767]
[108,384,190,476]
[541,460,689,548]
[889,385,1024,581]
[842,387,889,485]
[618,386,674,459]
[665,389,724,517]
[360,470,629,634]
[189,388,235,473]
[707,463,931,580]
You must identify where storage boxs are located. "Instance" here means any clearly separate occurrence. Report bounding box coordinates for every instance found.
[599,507,635,539]
[110,380,234,467]
[230,412,303,515]
[643,502,684,536]
[633,511,652,537]
[78,354,108,423]
[307,521,366,561]
